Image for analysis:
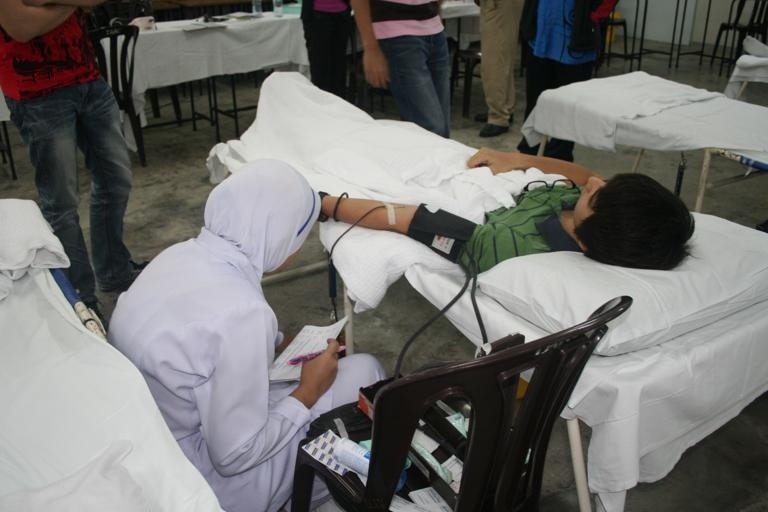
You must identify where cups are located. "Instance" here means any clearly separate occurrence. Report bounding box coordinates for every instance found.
[251,0,264,16]
[273,0,284,16]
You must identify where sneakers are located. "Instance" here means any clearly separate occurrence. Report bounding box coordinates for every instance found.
[80,294,109,334]
[479,122,510,138]
[475,112,514,124]
[95,258,150,293]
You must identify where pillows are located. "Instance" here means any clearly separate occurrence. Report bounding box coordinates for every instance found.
[469,211,767,356]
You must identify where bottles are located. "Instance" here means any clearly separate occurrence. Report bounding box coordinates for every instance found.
[333,437,408,493]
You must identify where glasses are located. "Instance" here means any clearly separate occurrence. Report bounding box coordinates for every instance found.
[517,179,582,204]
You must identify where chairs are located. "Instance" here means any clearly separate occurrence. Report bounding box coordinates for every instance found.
[598,15,627,66]
[290,294,633,511]
[711,0,768,88]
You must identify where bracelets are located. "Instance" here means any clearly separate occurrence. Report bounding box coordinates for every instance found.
[331,191,350,223]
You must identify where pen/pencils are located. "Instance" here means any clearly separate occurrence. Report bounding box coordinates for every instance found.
[287,345,348,365]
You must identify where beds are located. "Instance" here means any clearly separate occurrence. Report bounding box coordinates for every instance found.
[206,69,767,511]
[520,70,767,213]
[724,54,768,100]
[0,197,225,511]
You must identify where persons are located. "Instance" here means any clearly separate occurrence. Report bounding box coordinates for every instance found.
[249,67,696,279]
[351,1,453,138]
[516,1,620,164]
[474,1,525,135]
[104,158,387,512]
[1,0,152,336]
[301,1,354,98]
